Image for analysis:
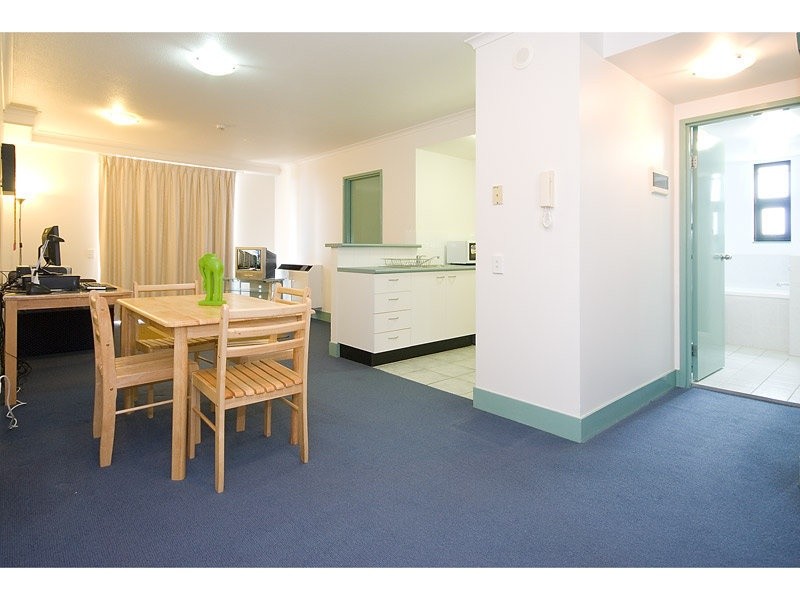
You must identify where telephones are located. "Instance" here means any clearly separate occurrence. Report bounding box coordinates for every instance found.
[26,282,51,294]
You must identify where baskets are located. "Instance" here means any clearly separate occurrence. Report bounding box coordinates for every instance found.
[383,258,432,268]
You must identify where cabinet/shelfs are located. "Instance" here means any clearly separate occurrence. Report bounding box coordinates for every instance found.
[339,270,476,366]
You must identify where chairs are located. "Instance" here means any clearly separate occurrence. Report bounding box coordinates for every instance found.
[89,281,313,494]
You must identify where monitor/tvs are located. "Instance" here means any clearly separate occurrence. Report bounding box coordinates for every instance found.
[34,225,62,275]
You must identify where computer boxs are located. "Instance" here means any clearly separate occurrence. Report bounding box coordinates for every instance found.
[20,273,80,292]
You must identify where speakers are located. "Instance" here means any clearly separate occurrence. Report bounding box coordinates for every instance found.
[234,246,277,280]
[1,144,16,192]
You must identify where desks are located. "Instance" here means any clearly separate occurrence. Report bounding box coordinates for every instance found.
[116,294,309,480]
[325,242,422,357]
[2,282,133,403]
[224,278,283,302]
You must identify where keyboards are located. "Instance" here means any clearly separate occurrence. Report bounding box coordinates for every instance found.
[81,282,107,289]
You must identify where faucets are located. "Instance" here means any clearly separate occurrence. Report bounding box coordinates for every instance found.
[416,254,440,267]
